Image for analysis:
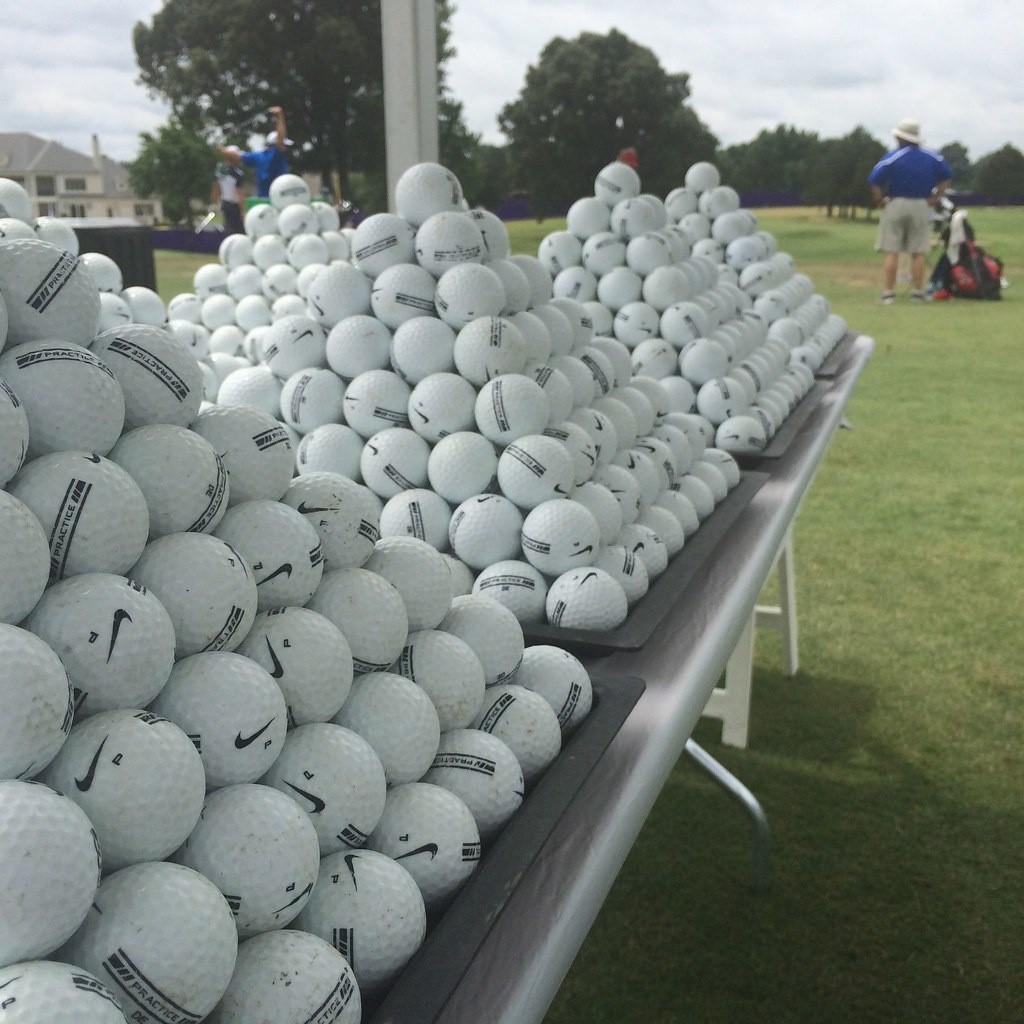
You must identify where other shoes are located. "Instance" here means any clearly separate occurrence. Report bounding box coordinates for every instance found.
[882,295,897,305]
[911,294,933,303]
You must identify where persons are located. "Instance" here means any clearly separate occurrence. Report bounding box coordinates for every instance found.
[217,106,287,201]
[866,120,952,309]
[208,144,247,234]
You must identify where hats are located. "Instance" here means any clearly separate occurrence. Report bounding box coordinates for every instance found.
[266,132,294,146]
[227,145,246,156]
[891,121,924,144]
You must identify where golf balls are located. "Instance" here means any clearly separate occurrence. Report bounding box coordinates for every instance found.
[0,161,593,1024]
[586,159,849,634]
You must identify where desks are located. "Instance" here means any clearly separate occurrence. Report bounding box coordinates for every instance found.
[359,331,875,1024]
